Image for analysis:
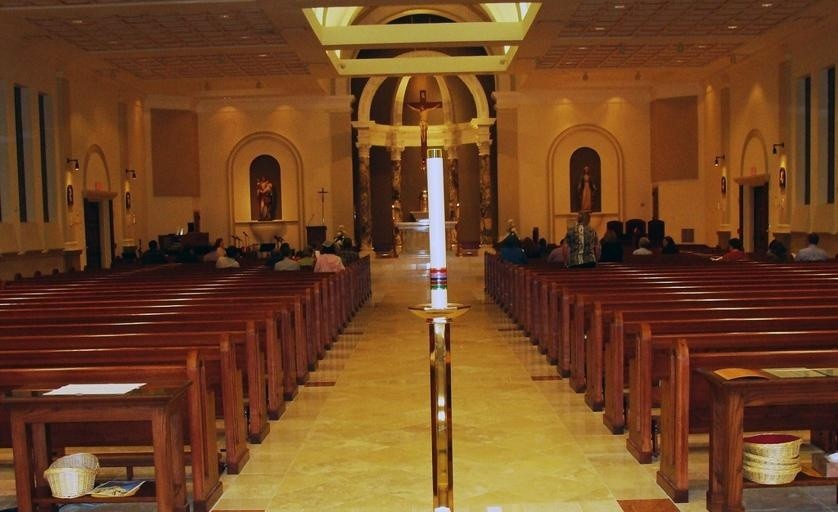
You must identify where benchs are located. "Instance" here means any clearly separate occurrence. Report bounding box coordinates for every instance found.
[484,243,838,512]
[0,253,372,512]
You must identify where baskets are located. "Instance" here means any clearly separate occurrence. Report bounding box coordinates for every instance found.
[43,453,99,498]
[743,435,803,485]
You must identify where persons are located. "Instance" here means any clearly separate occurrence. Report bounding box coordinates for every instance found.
[142,240,165,265]
[257,175,272,221]
[204,238,240,268]
[274,243,300,271]
[298,232,359,272]
[495,211,680,268]
[577,166,597,212]
[710,238,750,261]
[765,232,828,261]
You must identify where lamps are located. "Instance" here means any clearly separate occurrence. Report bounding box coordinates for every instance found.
[773,142,784,154]
[66,158,79,171]
[715,154,725,167]
[126,169,137,179]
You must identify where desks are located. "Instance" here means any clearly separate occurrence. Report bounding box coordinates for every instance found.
[395,221,459,256]
